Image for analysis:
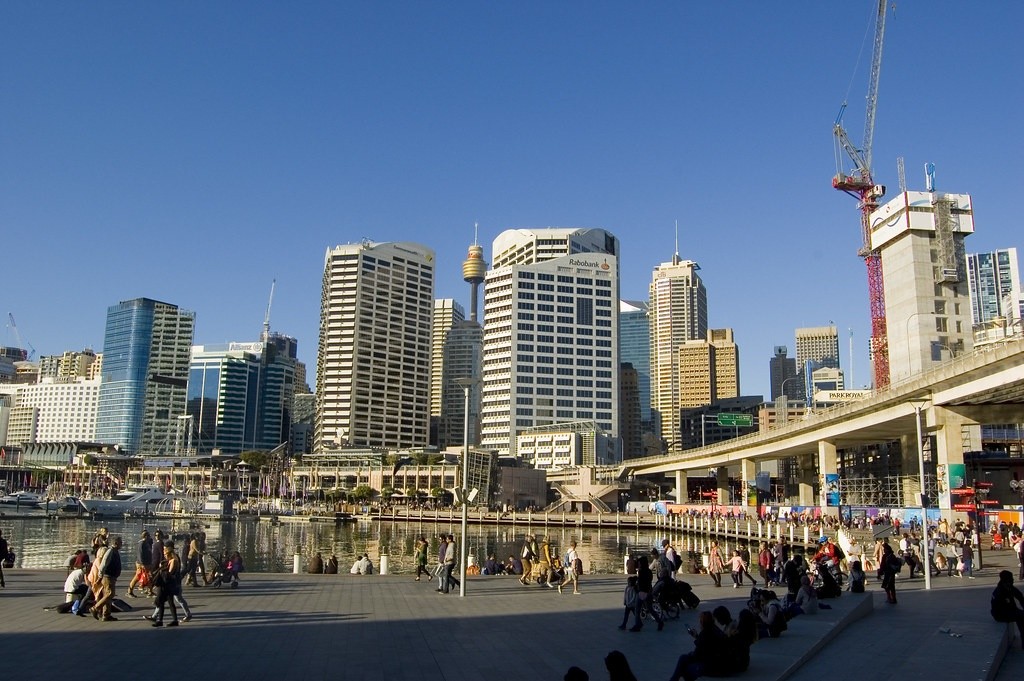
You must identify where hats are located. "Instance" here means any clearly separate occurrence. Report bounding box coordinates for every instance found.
[163,541,175,549]
[662,540,670,546]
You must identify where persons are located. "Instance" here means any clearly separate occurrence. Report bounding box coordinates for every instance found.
[180,532,245,587]
[350,553,373,575]
[306,552,338,574]
[991,570,1024,650]
[125,530,192,627]
[563,666,589,681]
[708,533,976,604]
[485,533,582,594]
[489,503,541,517]
[755,510,902,537]
[452,505,479,513]
[624,508,752,527]
[909,516,977,547]
[415,534,460,594]
[669,577,818,681]
[0,530,15,588]
[989,521,1024,581]
[604,650,638,681]
[292,496,446,511]
[63,528,122,621]
[618,539,676,633]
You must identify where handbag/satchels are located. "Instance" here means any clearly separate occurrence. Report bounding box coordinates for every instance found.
[138,569,152,588]
[71,596,80,615]
[764,603,788,639]
[894,557,901,572]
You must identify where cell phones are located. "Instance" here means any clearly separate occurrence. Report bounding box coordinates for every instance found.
[685,624,692,632]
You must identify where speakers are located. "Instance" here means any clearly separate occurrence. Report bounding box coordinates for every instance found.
[467,488,478,502]
[453,486,462,501]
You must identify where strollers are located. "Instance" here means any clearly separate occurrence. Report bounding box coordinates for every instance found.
[538,561,566,586]
[206,552,240,590]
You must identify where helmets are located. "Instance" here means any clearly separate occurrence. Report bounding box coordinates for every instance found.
[819,536,828,543]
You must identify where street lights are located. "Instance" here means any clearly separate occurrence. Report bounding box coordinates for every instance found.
[907,311,937,377]
[933,342,955,361]
[904,397,933,589]
[451,376,481,597]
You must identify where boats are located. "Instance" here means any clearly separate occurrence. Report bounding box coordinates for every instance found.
[0,485,177,517]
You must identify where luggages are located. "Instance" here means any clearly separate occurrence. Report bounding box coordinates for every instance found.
[681,590,700,609]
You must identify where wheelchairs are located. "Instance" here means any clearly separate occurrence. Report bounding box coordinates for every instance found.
[640,591,680,621]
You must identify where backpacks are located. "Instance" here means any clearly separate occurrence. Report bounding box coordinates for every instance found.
[665,547,682,571]
[91,535,100,554]
[834,545,845,559]
[851,571,864,593]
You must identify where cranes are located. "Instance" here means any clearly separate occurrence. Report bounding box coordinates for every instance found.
[262,279,278,336]
[829,0,895,390]
[28,342,38,361]
[8,311,25,361]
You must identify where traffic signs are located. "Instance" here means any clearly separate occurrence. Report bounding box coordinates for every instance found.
[952,478,999,511]
[717,413,754,426]
[978,512,999,516]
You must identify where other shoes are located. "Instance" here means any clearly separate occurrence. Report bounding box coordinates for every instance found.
[143,614,191,627]
[519,578,581,595]
[125,587,157,599]
[618,626,626,630]
[89,608,118,622]
[183,574,212,587]
[715,579,786,589]
[638,619,643,627]
[908,570,975,580]
[414,577,460,594]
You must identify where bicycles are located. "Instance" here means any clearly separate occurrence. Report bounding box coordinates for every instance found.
[805,561,851,593]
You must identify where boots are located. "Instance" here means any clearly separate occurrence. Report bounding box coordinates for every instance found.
[654,616,664,631]
[630,620,640,632]
